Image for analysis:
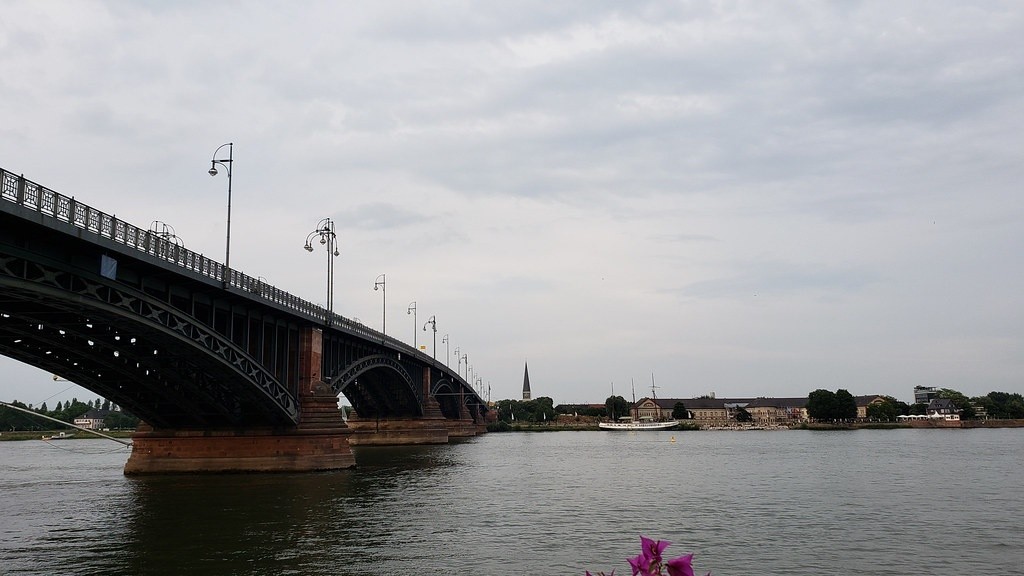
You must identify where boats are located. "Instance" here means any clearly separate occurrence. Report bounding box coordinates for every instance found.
[598,372,680,430]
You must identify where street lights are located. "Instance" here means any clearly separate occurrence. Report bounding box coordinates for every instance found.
[208,142,234,267]
[407,302,416,348]
[442,333,449,368]
[304,217,340,313]
[454,346,491,405]
[374,273,386,335]
[422,314,437,360]
